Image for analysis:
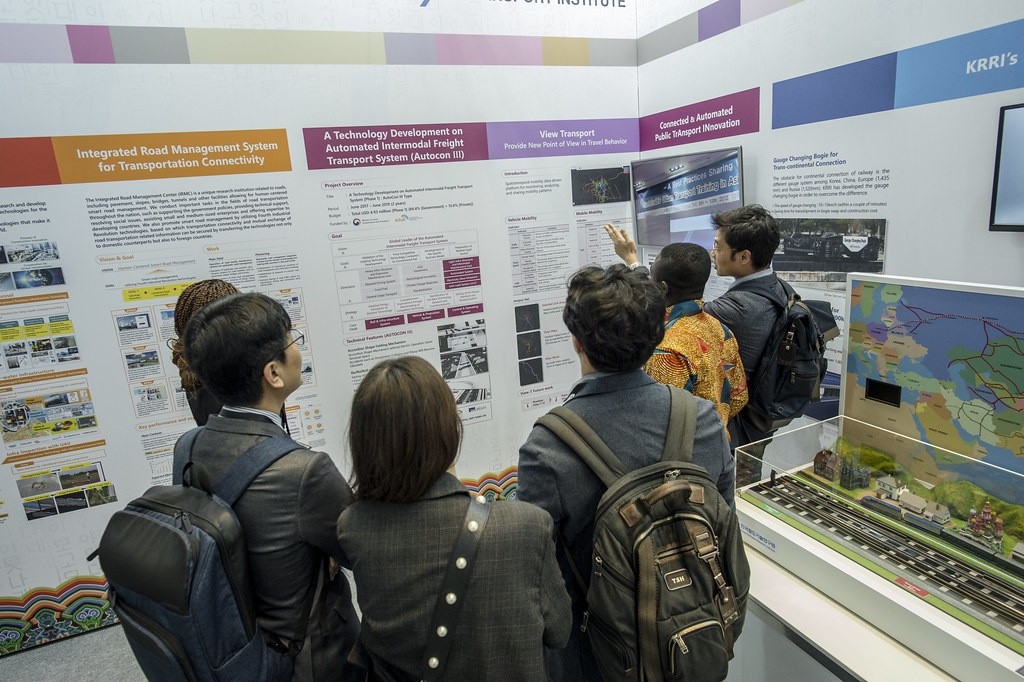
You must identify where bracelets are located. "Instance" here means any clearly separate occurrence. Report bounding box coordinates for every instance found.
[628,262,642,270]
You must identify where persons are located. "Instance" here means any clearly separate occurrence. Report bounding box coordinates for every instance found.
[604,203,796,487]
[639,242,749,444]
[169,278,355,682]
[335,357,572,682]
[516,263,735,682]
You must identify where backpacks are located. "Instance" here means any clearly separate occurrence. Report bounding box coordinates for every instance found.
[727,278,840,444]
[533,384,751,682]
[88,425,325,682]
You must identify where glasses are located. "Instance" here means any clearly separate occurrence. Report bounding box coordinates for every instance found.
[261,328,305,376]
[712,243,744,252]
